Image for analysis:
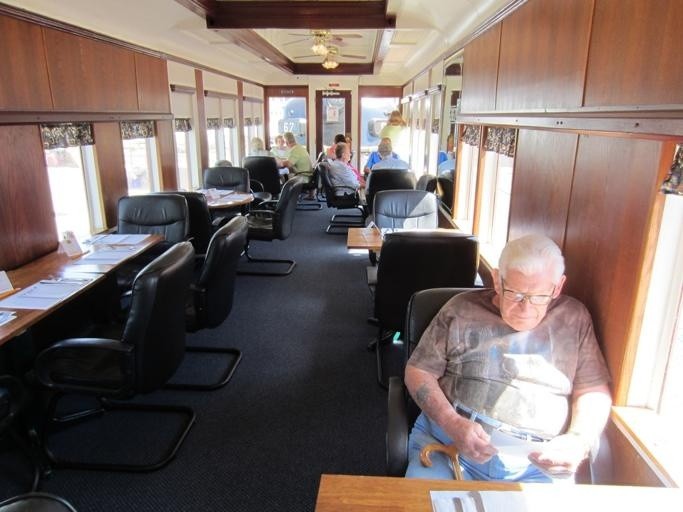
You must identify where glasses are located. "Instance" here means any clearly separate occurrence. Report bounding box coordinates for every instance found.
[500,278,557,305]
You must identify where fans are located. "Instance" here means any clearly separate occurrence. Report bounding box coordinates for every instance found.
[291,52,371,66]
[280,28,364,51]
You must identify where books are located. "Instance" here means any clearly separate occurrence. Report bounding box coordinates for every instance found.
[0,309,17,327]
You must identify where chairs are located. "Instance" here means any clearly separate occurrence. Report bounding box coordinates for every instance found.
[109,214,250,394]
[105,193,190,260]
[365,228,482,388]
[316,153,446,237]
[183,146,323,278]
[386,286,597,483]
[34,240,199,486]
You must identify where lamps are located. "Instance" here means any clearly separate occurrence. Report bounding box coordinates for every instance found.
[384,110,409,131]
[322,57,344,77]
[311,38,331,58]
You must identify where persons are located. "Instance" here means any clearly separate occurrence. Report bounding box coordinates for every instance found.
[400,231,617,484]
[212,159,232,168]
[245,130,364,202]
[376,110,408,147]
[362,137,403,176]
[364,143,414,196]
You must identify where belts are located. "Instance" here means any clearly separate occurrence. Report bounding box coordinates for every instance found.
[456,406,551,444]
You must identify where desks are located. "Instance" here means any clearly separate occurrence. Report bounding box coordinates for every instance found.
[1,229,168,344]
[345,225,477,253]
[307,464,683,512]
